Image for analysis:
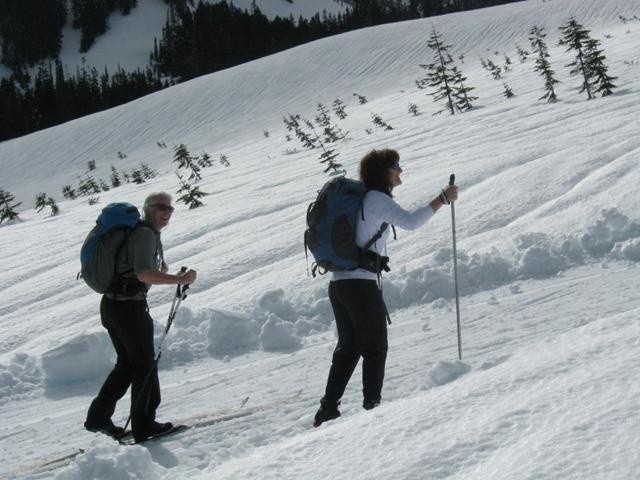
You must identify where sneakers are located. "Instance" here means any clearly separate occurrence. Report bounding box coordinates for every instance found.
[84,418,125,436]
[134,421,173,442]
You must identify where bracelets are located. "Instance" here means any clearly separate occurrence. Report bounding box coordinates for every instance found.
[438,190,451,205]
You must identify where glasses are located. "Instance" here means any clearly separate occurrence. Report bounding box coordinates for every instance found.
[391,163,399,169]
[148,204,174,212]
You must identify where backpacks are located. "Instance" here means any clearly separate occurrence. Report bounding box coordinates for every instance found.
[303,177,392,272]
[80,202,151,295]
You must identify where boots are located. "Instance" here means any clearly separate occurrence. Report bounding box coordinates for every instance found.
[313,398,342,426]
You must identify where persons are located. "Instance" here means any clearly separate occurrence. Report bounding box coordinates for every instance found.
[310,149,458,426]
[84,191,197,445]
